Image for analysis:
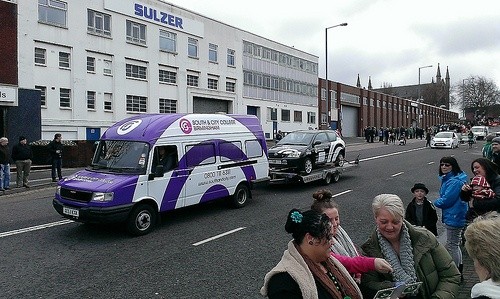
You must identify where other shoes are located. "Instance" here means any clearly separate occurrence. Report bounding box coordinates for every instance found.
[0,188,5,191]
[4,187,11,190]
[23,183,31,188]
[16,185,21,188]
[52,179,58,182]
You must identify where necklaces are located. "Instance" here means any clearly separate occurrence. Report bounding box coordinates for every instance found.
[328,271,339,291]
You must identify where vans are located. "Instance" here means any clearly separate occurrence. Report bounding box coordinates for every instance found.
[52,113,270,236]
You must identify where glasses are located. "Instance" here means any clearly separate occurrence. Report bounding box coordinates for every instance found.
[441,163,449,167]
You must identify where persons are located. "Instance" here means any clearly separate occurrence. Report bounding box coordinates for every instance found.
[260,208,365,299]
[434,155,470,283]
[404,183,439,237]
[482,134,500,175]
[468,129,474,148]
[310,188,393,285]
[461,157,500,219]
[275,130,284,144]
[378,126,425,144]
[364,125,376,143]
[48,133,65,182]
[471,175,496,199]
[358,193,461,299]
[425,133,432,147]
[0,137,13,191]
[464,211,500,299]
[13,136,33,188]
[156,147,171,173]
[426,122,471,136]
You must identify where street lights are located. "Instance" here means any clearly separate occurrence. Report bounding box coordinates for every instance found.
[418,65,432,127]
[463,78,474,117]
[325,22,347,130]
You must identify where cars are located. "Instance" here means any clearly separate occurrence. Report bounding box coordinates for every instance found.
[267,129,346,174]
[430,125,488,149]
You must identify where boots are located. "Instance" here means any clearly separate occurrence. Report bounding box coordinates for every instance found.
[458,263,464,285]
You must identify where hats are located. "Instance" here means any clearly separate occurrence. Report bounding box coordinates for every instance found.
[492,137,500,143]
[18,136,26,142]
[411,183,429,194]
